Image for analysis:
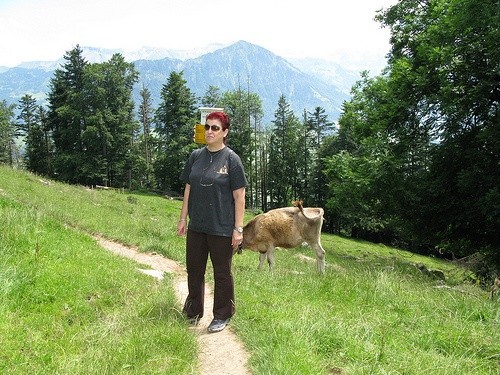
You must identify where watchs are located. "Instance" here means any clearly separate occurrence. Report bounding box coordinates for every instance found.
[234,226,244,233]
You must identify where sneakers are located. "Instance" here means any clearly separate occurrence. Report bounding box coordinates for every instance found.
[184,317,230,332]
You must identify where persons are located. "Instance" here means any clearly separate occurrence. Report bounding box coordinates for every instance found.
[177,111,247,332]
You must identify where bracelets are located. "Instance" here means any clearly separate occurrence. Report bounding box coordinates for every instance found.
[179,218,185,222]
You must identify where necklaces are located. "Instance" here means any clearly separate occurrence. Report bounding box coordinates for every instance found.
[199,144,228,187]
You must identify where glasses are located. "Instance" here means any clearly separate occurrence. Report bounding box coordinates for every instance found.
[205,124,220,131]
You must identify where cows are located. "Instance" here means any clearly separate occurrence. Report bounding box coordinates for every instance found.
[232,199,327,274]
